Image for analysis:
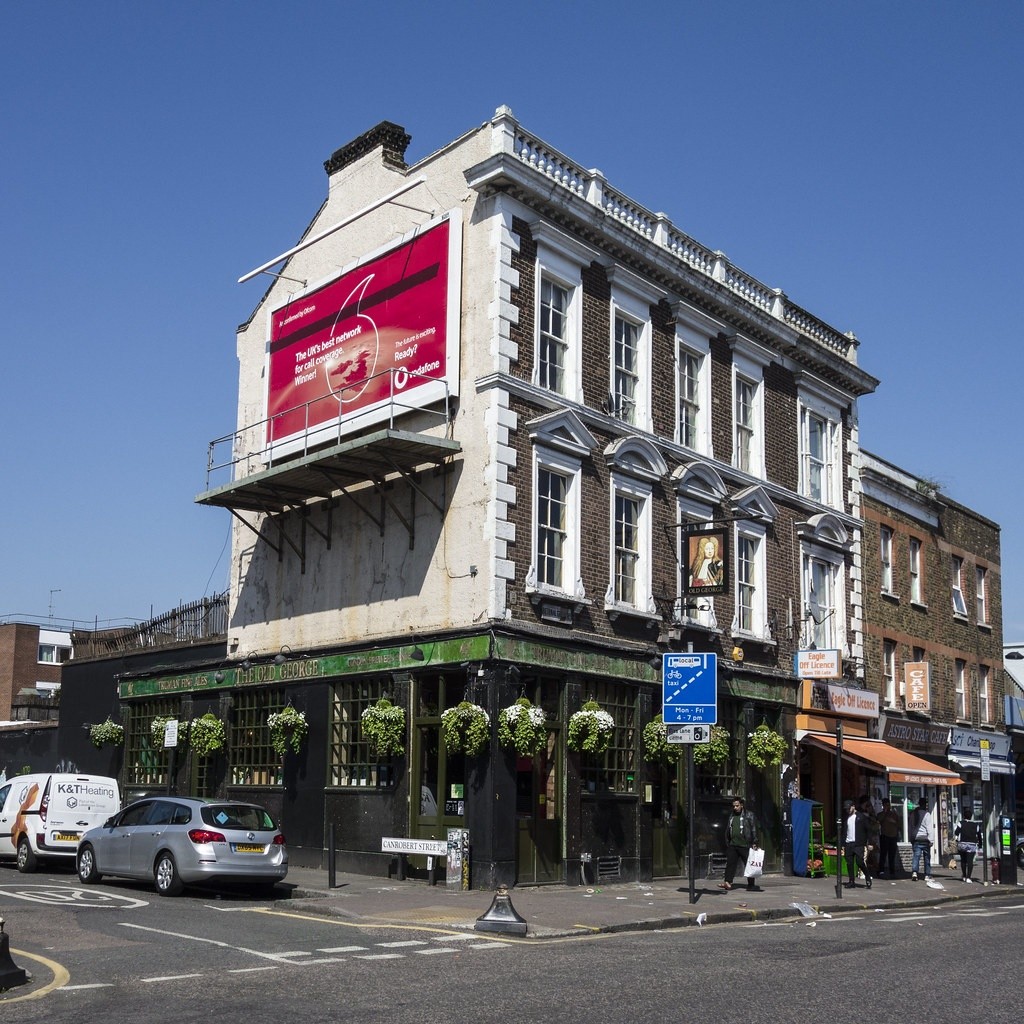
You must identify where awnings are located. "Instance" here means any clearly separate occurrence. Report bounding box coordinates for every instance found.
[947,753,1016,775]
[796,732,964,784]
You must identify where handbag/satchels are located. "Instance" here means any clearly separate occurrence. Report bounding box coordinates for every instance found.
[743,847,765,877]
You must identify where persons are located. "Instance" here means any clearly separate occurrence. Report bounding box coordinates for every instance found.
[716,798,761,892]
[907,798,935,881]
[855,795,898,879]
[840,801,874,889]
[954,810,982,883]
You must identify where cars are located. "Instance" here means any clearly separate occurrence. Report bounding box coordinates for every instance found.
[74,796,289,898]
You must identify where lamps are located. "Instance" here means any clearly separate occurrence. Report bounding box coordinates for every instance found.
[275,645,296,666]
[242,651,259,671]
[718,662,734,680]
[411,633,438,661]
[216,662,240,685]
[628,646,662,672]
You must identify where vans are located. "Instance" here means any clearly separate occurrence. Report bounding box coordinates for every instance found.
[0,772,120,873]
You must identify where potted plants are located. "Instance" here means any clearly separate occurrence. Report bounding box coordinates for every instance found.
[746,726,788,770]
[567,696,614,754]
[440,701,490,756]
[90,719,124,752]
[362,699,405,756]
[151,716,188,753]
[693,726,730,766]
[498,699,547,756]
[642,715,682,763]
[268,707,307,758]
[190,713,226,758]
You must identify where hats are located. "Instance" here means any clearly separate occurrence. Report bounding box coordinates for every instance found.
[843,800,852,808]
[918,798,928,804]
[858,795,870,804]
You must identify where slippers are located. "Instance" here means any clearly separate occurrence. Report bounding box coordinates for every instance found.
[746,884,755,890]
[717,882,732,890]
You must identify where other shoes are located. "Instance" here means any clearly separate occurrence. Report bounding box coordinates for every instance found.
[845,881,855,888]
[890,874,896,879]
[866,876,872,887]
[925,876,935,882]
[966,878,972,883]
[963,877,966,881]
[879,872,885,878]
[912,872,918,881]
